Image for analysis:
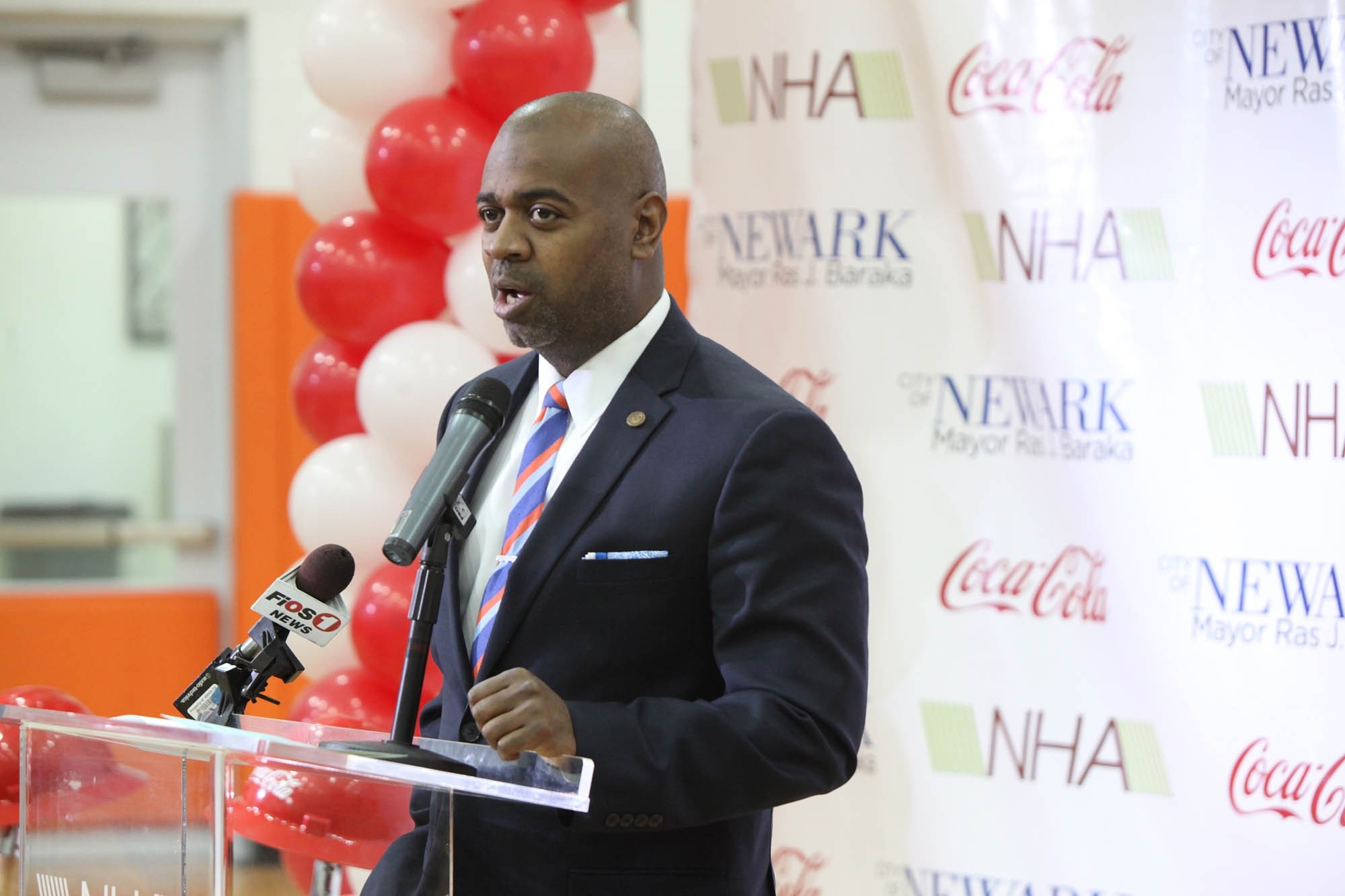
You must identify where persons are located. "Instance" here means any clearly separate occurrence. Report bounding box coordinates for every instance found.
[361,91,871,896]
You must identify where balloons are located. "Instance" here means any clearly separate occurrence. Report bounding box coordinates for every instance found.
[246,0,618,841]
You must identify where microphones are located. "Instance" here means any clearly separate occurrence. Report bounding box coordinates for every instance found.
[222,544,356,668]
[382,375,516,565]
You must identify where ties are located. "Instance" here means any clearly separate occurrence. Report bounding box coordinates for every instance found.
[469,379,568,681]
